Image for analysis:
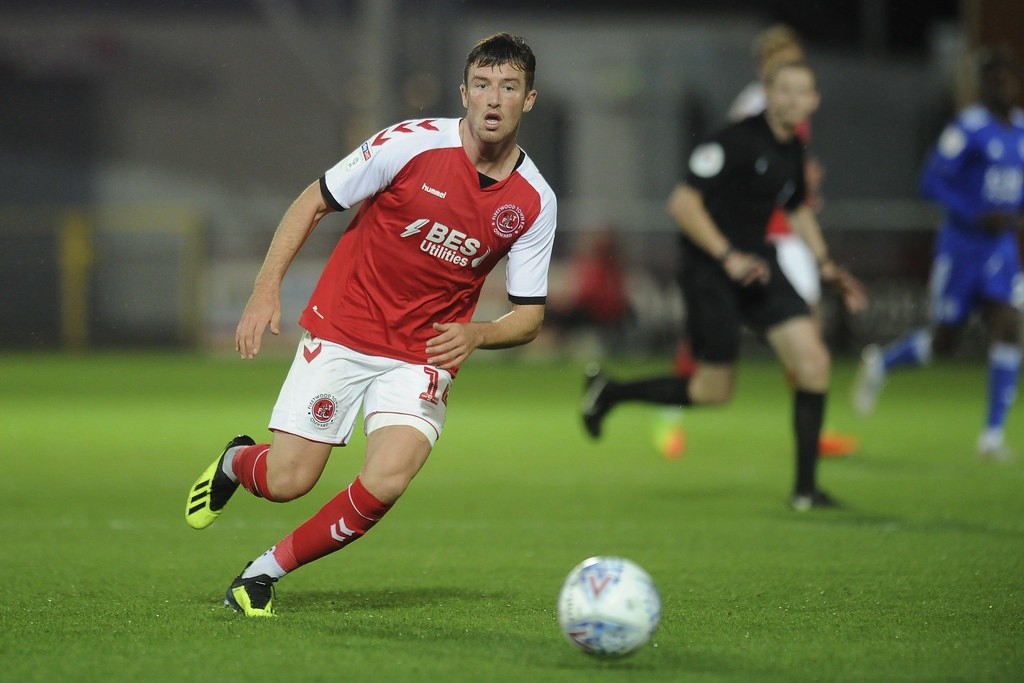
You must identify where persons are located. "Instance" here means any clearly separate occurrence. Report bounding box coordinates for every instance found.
[653,26,864,458]
[184,32,559,618]
[585,61,841,508]
[852,56,1023,454]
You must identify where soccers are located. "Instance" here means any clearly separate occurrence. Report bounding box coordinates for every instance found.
[558,555,661,657]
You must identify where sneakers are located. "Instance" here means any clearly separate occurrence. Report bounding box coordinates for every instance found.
[789,489,835,509]
[584,371,613,439]
[224,561,279,617]
[185,435,256,530]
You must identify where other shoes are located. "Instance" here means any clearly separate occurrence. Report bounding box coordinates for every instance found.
[980,432,1015,466]
[851,349,884,413]
[657,407,688,457]
[818,430,852,456]
